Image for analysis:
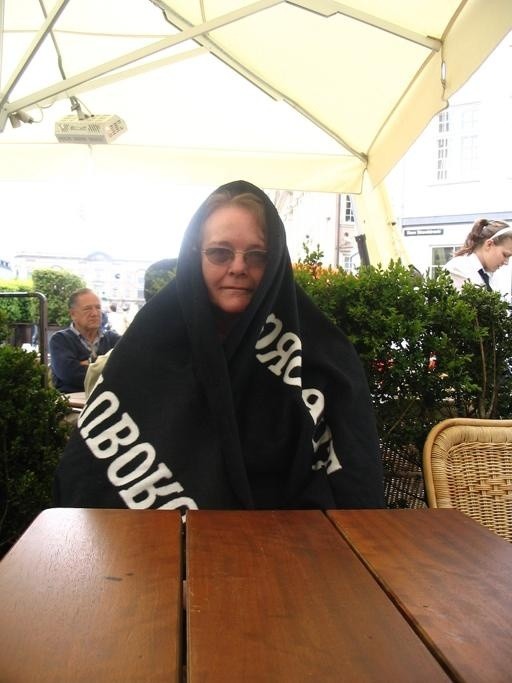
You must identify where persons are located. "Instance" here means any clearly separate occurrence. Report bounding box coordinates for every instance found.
[48,286,122,394]
[49,179,389,617]
[434,216,511,314]
[108,302,125,334]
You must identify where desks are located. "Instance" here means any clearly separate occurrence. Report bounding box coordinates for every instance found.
[0,508,512,682]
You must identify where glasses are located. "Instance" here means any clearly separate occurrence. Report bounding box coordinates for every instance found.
[200,246,267,268]
